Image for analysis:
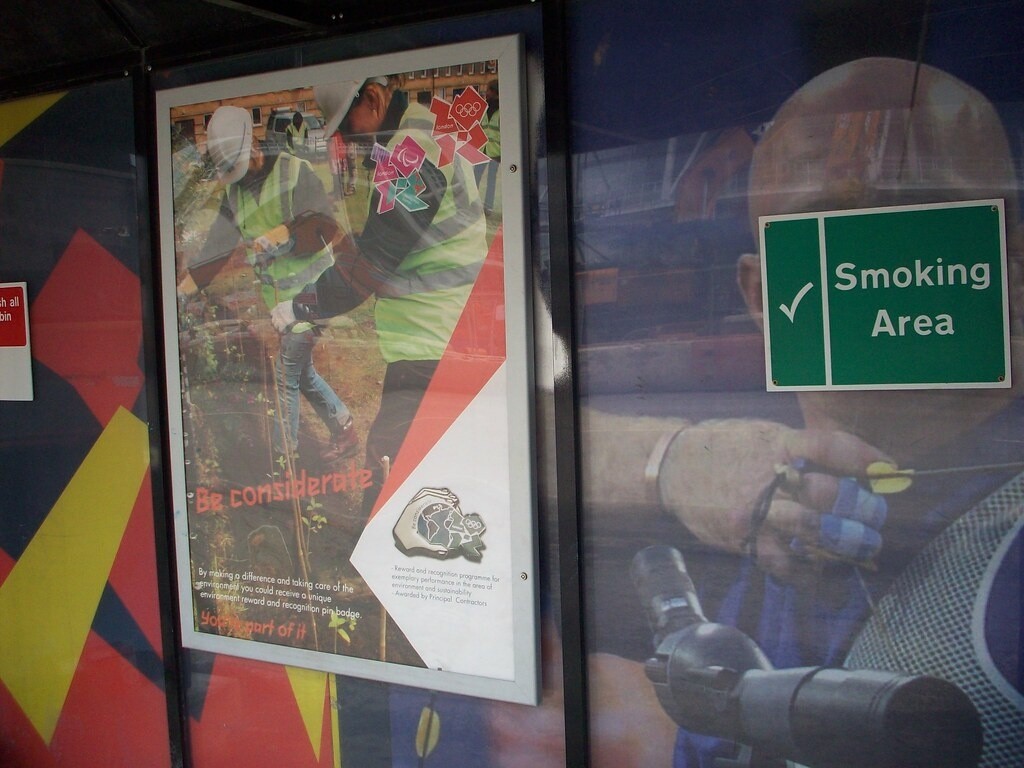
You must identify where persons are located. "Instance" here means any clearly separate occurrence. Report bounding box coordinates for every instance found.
[166,47,1024,768]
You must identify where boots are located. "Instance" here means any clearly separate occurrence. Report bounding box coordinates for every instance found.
[344,168,358,195]
[328,172,347,200]
[318,415,362,466]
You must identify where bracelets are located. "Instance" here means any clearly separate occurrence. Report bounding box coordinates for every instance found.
[644,416,695,520]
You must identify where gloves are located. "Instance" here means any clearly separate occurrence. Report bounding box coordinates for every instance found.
[269,300,296,333]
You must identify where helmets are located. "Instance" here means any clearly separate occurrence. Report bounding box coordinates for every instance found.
[313,77,371,142]
[205,105,254,184]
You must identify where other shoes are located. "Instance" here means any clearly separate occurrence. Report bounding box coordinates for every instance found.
[484,205,495,217]
